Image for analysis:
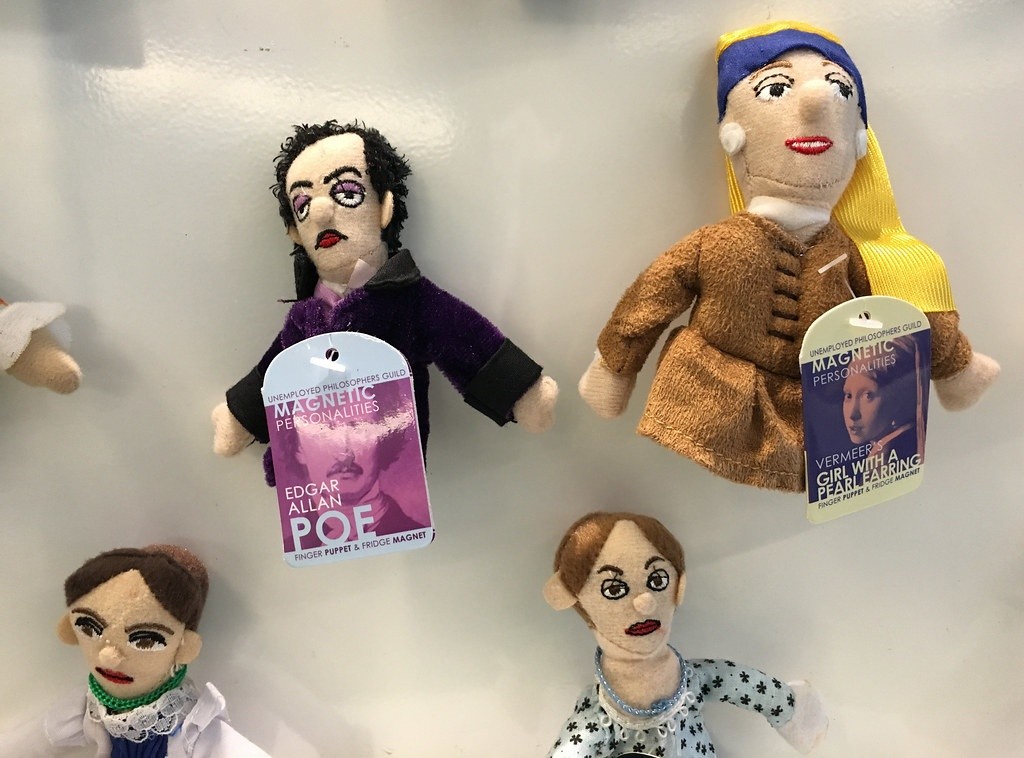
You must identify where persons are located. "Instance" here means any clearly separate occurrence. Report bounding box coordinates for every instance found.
[843,335,926,484]
[277,381,426,552]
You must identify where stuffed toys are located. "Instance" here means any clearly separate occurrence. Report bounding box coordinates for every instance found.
[0,298,81,396]
[46,543,271,758]
[577,20,1001,494]
[211,119,557,488]
[543,513,831,758]
